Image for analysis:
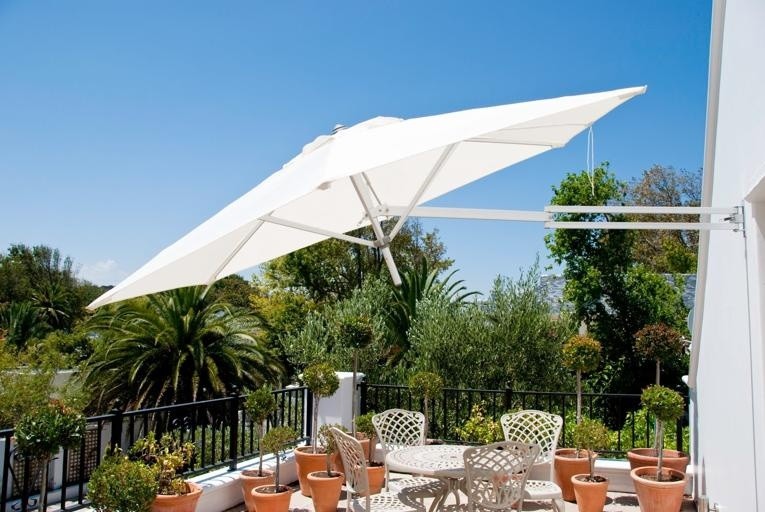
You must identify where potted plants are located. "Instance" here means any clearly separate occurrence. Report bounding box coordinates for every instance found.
[629,383,689,512]
[307,424,347,512]
[352,410,384,499]
[627,323,689,474]
[250,426,300,512]
[553,335,604,502]
[333,317,374,487]
[239,383,280,512]
[127,430,203,512]
[294,361,337,496]
[564,415,611,512]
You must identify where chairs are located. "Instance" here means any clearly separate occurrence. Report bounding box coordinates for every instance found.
[328,427,426,512]
[371,408,460,512]
[500,410,565,511]
[462,442,541,512]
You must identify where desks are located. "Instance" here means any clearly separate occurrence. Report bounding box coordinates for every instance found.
[385,445,523,512]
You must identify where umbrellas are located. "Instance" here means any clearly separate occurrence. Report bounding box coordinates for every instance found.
[84,82,650,313]
